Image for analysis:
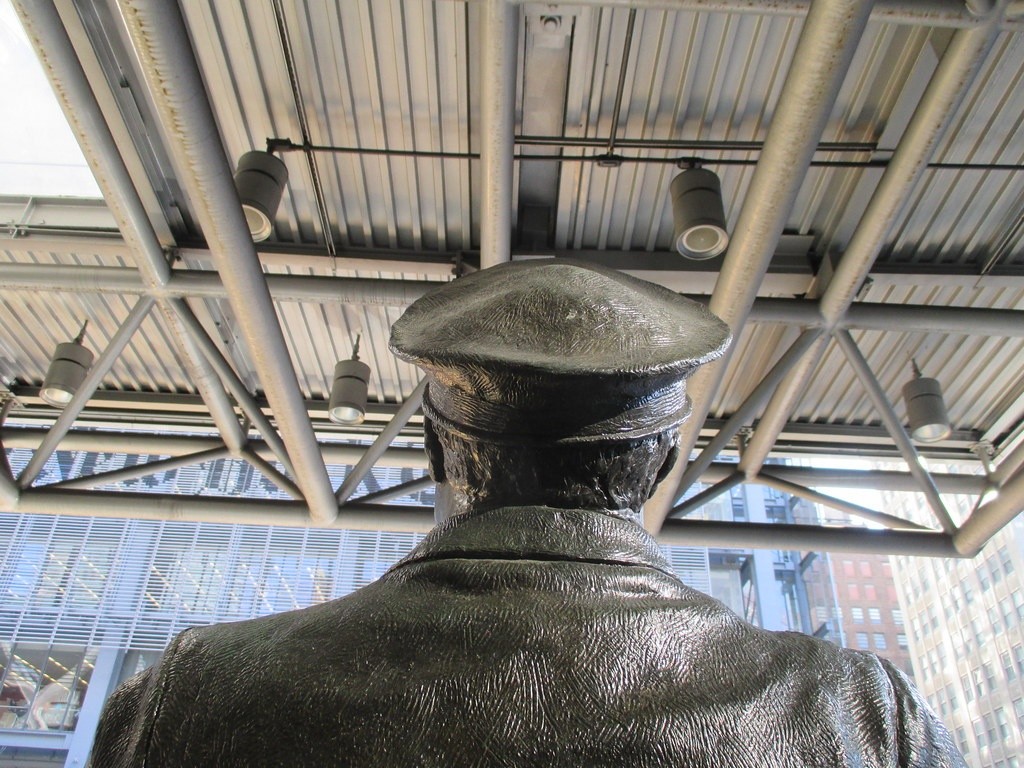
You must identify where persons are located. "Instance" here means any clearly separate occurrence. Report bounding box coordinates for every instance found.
[84,257,969,768]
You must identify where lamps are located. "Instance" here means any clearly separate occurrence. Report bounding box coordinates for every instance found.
[902,356,952,444]
[40,319,94,406]
[670,159,730,261]
[232,140,289,241]
[327,334,373,426]
